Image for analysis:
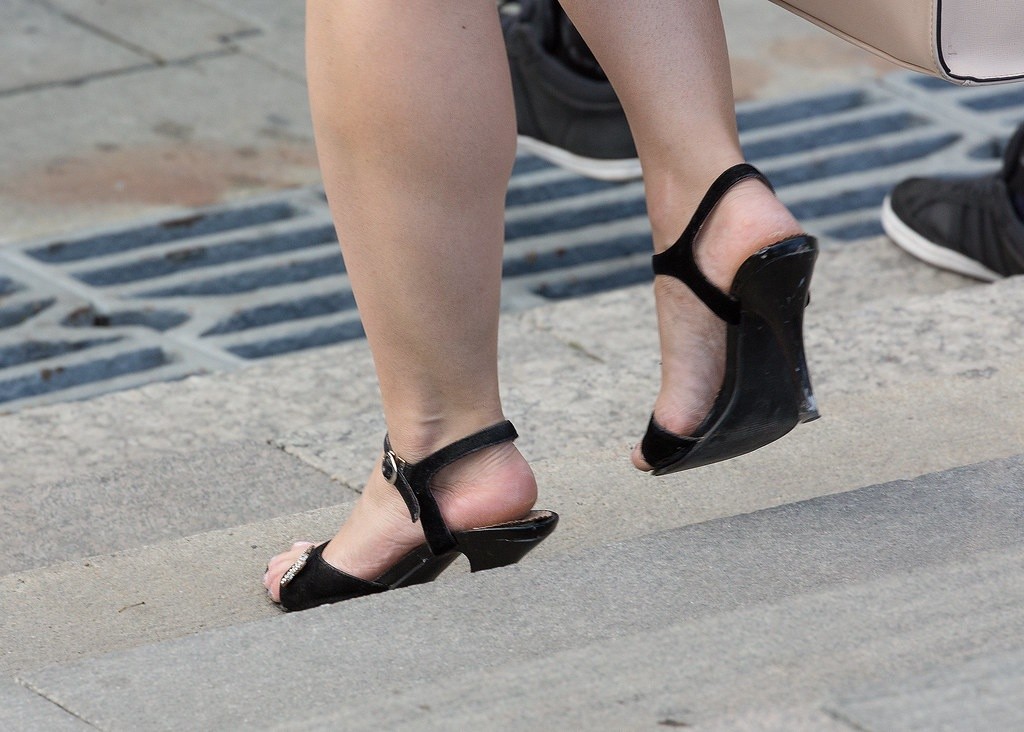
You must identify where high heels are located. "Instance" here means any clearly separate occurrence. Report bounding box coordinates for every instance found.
[641,163,819,476]
[263,417,556,616]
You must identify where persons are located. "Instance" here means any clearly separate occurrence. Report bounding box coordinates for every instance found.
[876,121,1024,284]
[260,2,820,614]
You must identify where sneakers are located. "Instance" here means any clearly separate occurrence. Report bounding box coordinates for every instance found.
[498,0,642,181]
[881,175,1024,282]
